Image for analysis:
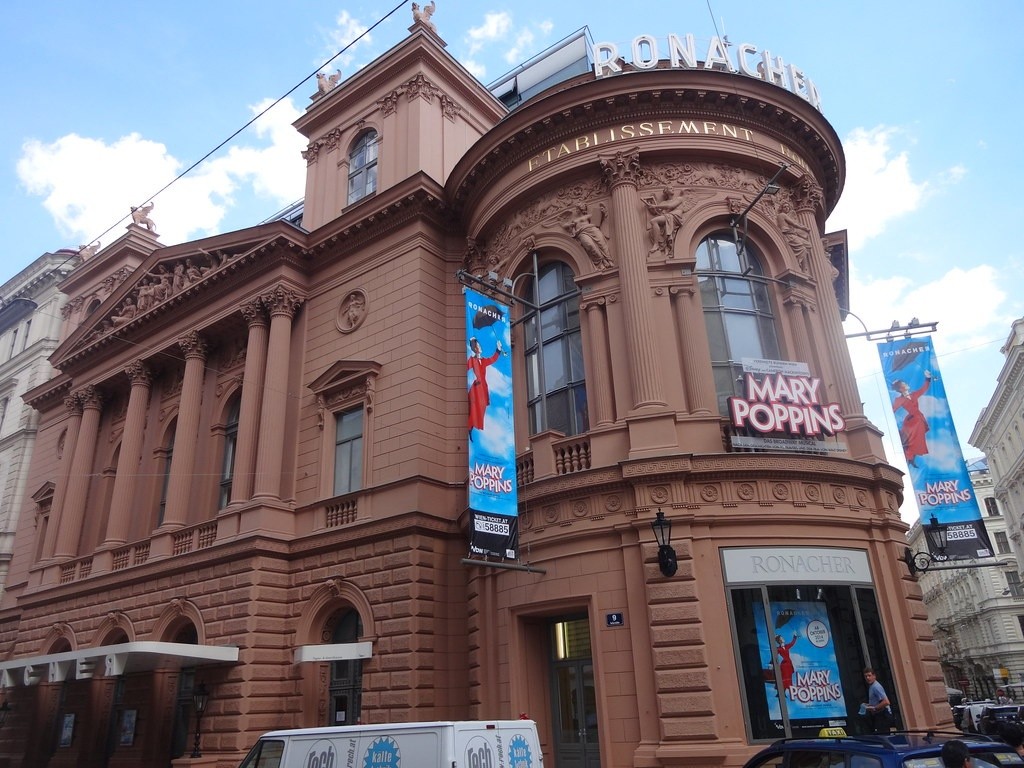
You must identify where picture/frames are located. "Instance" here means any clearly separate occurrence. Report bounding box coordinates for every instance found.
[120,708,138,747]
[59,712,76,748]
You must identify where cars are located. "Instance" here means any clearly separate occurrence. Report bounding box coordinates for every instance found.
[943,686,1024,738]
[742,725,1021,768]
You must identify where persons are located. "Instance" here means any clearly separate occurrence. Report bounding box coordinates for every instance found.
[861,667,894,734]
[960,693,973,706]
[998,722,1024,758]
[773,630,800,701]
[890,369,934,468]
[466,337,503,442]
[941,740,972,768]
[994,690,1014,705]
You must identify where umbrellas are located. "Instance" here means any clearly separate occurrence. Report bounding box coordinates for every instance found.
[892,342,938,383]
[471,305,509,357]
[775,608,802,639]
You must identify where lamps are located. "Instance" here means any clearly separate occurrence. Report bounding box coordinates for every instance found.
[904,513,948,577]
[1001,575,1021,595]
[960,621,966,627]
[489,270,515,290]
[648,505,679,577]
[192,681,209,718]
[0,699,11,729]
[762,181,781,194]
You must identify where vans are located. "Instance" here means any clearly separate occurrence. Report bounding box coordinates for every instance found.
[237,717,545,768]
